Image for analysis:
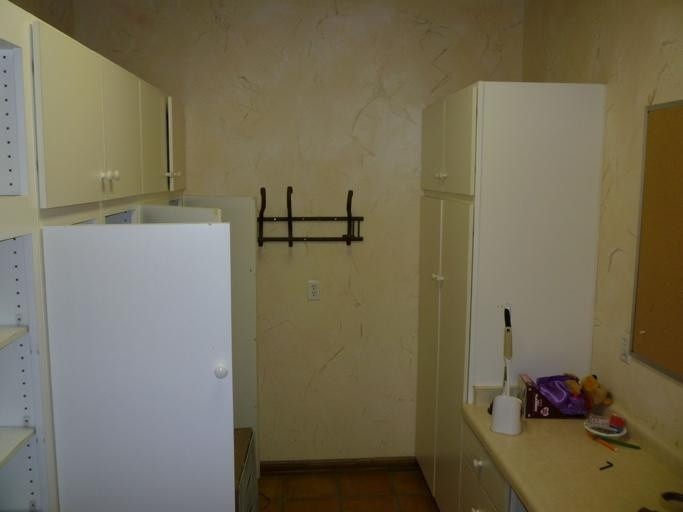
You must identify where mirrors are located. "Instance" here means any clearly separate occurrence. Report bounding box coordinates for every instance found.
[626,97,683,387]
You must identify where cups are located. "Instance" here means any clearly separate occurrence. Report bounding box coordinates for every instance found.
[491,395,523,435]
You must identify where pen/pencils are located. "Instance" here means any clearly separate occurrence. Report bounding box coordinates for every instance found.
[594,437,642,452]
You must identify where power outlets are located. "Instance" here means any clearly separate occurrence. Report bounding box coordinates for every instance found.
[307,280,320,301]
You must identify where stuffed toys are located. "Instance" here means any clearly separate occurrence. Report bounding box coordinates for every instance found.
[563,372,614,412]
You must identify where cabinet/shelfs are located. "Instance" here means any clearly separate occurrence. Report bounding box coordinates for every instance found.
[0,0,258,512]
[422,80,605,195]
[456,420,508,512]
[417,196,602,512]
